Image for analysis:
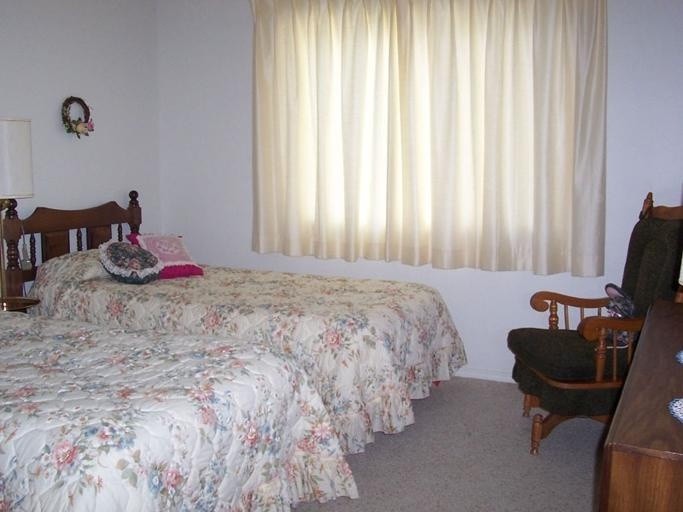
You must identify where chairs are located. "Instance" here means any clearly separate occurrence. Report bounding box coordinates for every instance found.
[507,193,682,454]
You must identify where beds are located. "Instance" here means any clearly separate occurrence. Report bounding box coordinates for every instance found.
[0,308,363,510]
[4,190,467,456]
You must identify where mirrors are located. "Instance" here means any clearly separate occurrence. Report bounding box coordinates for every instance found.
[61,96,94,136]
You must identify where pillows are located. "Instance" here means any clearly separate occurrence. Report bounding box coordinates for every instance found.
[598,283,643,350]
[97,237,164,286]
[124,232,205,279]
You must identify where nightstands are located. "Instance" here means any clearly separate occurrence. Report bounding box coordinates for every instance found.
[0,295,42,315]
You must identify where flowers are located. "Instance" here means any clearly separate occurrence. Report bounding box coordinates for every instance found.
[71,117,95,137]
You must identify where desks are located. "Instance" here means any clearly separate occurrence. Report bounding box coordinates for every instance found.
[600,286,682,512]
